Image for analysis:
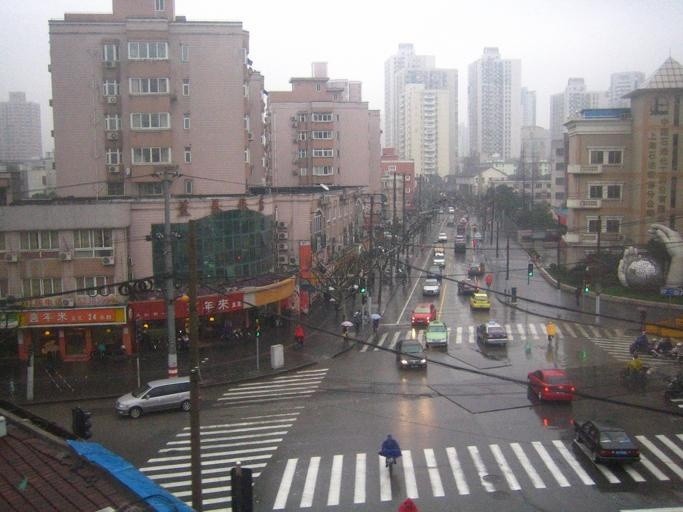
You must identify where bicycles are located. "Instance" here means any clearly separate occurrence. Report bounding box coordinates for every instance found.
[385,456,396,477]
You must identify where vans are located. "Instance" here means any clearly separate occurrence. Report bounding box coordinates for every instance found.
[113,375,192,419]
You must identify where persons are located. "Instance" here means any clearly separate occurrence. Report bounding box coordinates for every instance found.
[327,257,410,335]
[546,320,556,337]
[77,409,91,440]
[375,433,399,468]
[485,273,492,286]
[575,285,581,306]
[638,308,646,328]
[293,323,305,348]
[617,245,643,289]
[397,498,420,512]
[646,222,682,290]
[342,326,349,348]
[619,328,682,396]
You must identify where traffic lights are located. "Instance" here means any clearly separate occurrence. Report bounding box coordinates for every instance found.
[360,279,366,294]
[255,319,261,337]
[584,280,590,293]
[528,264,533,277]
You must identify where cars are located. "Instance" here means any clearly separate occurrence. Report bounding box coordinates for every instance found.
[457,262,491,311]
[438,233,447,242]
[423,243,445,296]
[574,418,640,466]
[447,206,481,252]
[527,368,576,403]
[477,320,509,345]
[396,302,448,371]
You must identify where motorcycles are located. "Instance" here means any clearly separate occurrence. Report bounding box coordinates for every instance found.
[621,337,683,415]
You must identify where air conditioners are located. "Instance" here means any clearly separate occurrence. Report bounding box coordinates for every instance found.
[108,97,117,104]
[61,298,74,307]
[58,251,72,261]
[109,166,120,173]
[4,254,18,262]
[107,132,119,140]
[101,256,115,266]
[277,222,296,266]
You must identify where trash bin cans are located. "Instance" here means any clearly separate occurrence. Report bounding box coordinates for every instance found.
[270,344,284,369]
